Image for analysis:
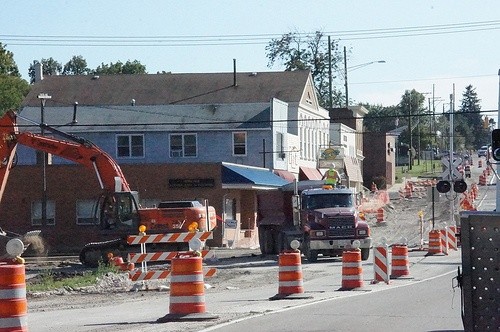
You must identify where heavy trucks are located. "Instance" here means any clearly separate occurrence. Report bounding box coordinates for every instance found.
[253,176,373,263]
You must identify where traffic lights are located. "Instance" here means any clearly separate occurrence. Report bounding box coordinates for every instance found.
[491,129,500,162]
[453,181,467,194]
[435,181,451,194]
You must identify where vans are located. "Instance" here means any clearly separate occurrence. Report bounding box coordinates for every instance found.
[477,145,488,157]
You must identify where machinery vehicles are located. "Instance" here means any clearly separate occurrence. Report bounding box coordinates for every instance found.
[0,106,220,269]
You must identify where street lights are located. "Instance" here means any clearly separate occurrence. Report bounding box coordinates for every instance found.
[329,60,386,110]
